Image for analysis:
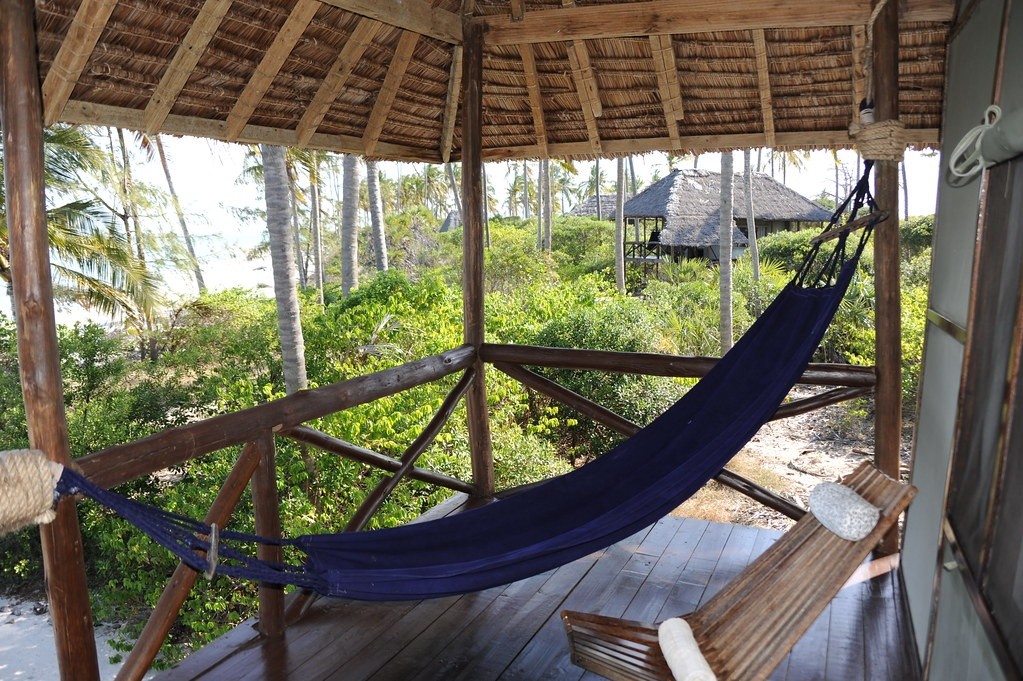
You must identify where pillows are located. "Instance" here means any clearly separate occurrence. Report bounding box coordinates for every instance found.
[657,616,717,681]
[806,479,880,541]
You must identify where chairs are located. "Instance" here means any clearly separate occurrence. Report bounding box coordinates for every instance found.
[561,459,919,681]
[625,231,665,259]
